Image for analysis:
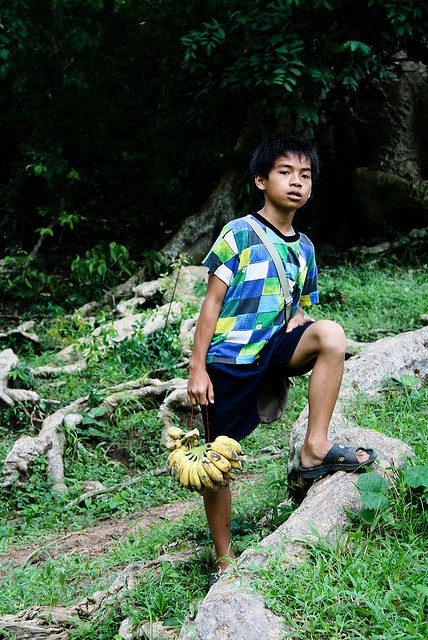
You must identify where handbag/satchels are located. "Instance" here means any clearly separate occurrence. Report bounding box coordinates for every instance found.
[243,214,294,422]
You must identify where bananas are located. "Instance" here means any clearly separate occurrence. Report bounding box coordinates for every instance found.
[167,426,244,493]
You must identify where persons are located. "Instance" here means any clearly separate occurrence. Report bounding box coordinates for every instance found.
[187,142,377,595]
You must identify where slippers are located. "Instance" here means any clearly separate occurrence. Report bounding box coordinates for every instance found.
[298,442,377,480]
